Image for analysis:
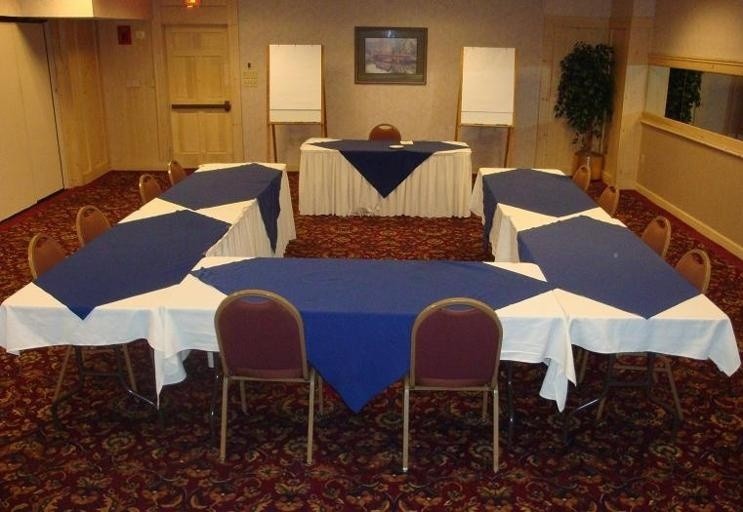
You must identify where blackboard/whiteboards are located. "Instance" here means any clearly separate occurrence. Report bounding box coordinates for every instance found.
[266,43,324,125]
[458,44,518,128]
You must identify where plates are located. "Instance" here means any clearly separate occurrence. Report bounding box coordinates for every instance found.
[390,144,404,149]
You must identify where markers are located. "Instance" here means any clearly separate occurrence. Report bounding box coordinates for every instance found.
[475,124,482,125]
[496,124,508,127]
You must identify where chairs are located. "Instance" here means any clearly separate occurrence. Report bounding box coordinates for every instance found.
[138,173,162,205]
[74,205,155,372]
[593,248,712,422]
[367,123,401,141]
[400,297,503,476]
[26,231,143,407]
[165,157,188,187]
[211,288,326,464]
[571,165,592,191]
[596,181,621,217]
[573,214,673,387]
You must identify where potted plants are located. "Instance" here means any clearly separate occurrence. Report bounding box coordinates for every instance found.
[554,40,618,179]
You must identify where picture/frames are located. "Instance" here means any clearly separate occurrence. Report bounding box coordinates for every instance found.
[352,25,428,85]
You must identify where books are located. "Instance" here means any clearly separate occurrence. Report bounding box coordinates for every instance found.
[400,140,413,145]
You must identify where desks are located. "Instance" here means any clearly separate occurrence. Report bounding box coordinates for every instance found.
[0,160,298,417]
[159,256,573,435]
[297,137,474,221]
[472,168,740,422]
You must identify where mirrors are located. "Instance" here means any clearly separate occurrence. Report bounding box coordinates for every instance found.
[639,52,742,160]
